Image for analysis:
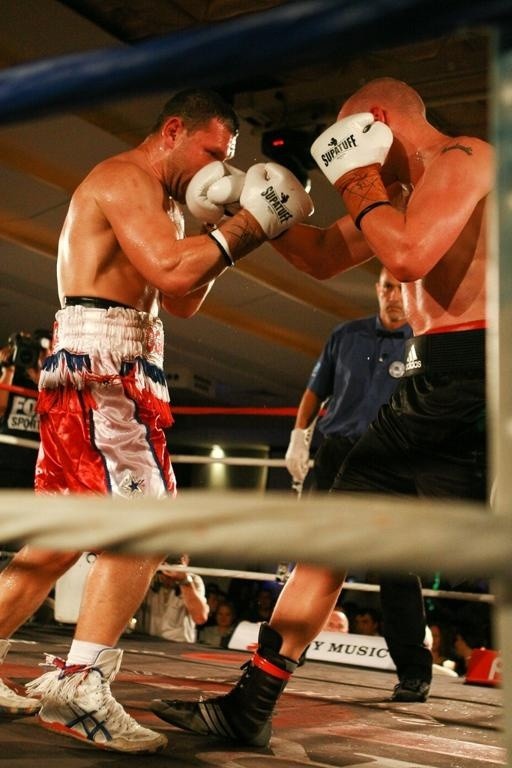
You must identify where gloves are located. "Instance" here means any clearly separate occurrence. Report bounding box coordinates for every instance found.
[185,160,314,268]
[284,428,312,481]
[309,111,395,230]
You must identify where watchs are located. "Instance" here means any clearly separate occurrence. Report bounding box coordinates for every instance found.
[178,575,194,584]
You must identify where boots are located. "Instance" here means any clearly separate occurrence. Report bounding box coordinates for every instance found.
[0,639,170,755]
[149,621,310,752]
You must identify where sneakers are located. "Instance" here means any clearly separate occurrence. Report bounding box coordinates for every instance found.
[390,677,431,702]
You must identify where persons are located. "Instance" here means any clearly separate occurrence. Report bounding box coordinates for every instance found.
[1,80,316,758]
[146,546,210,646]
[281,262,436,707]
[1,324,54,446]
[148,73,511,761]
[200,564,486,679]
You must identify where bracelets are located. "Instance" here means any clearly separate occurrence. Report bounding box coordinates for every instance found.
[208,228,236,268]
[354,200,392,230]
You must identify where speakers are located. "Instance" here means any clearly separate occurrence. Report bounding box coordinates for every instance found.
[259,125,322,172]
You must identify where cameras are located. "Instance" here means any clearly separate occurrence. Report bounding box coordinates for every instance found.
[163,553,184,565]
